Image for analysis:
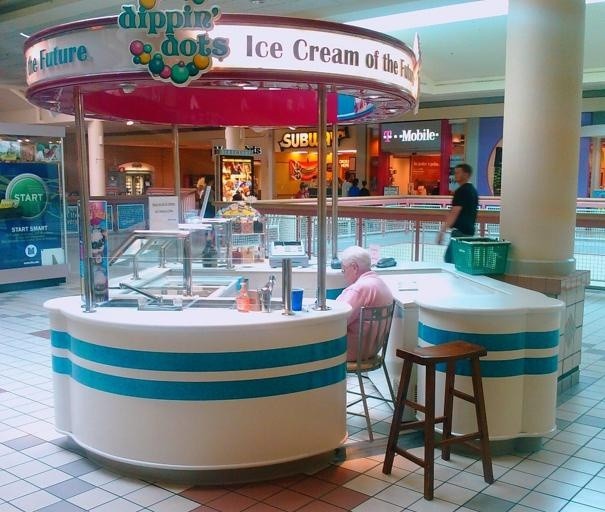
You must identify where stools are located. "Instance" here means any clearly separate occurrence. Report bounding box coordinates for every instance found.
[382,339,493,501]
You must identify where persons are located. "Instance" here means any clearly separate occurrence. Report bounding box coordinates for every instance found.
[331,246,394,362]
[36,145,57,161]
[295,182,309,199]
[436,164,478,263]
[196,175,215,218]
[341,173,370,196]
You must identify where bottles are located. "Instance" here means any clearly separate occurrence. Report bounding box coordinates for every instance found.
[236,276,250,313]
[202,238,218,268]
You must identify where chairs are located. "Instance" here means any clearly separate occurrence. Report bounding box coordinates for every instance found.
[342,298,398,440]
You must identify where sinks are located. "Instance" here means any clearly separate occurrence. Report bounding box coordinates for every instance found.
[147,297,283,311]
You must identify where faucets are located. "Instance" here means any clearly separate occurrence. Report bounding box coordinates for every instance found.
[119,281,162,305]
[261,274,277,311]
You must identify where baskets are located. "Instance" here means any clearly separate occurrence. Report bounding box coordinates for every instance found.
[450,236,512,275]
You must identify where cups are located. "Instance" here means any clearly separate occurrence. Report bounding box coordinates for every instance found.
[291,289,304,312]
